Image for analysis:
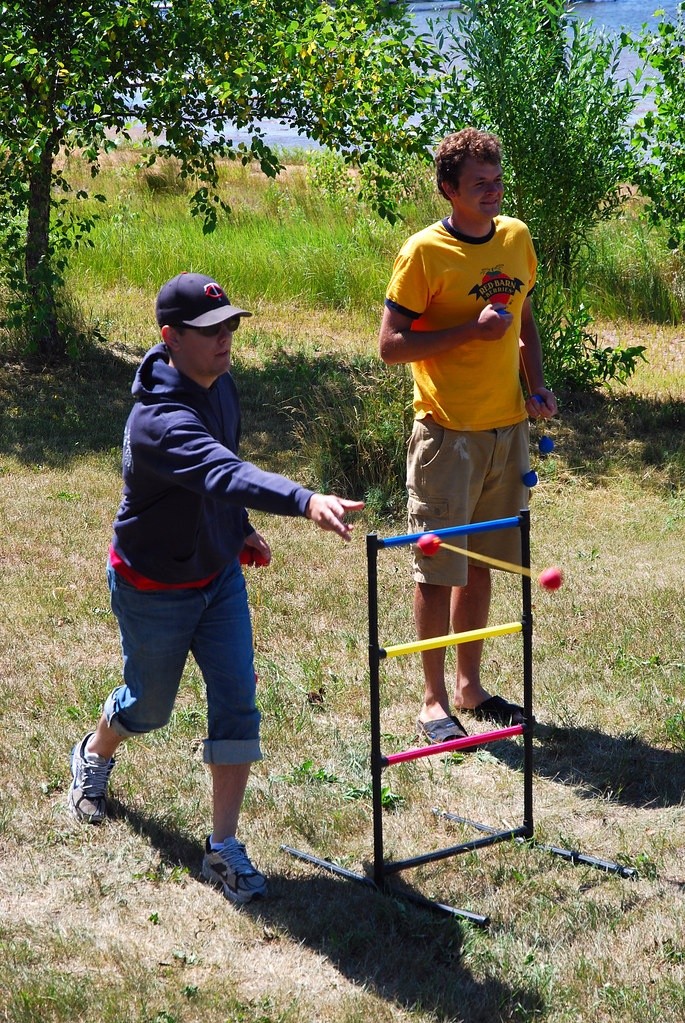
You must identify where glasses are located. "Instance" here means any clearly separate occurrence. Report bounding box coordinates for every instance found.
[182,313,240,337]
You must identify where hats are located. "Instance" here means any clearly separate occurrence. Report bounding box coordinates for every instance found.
[155,270,252,328]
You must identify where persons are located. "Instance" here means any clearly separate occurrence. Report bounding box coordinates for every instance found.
[69,270,366,904]
[379,127,558,752]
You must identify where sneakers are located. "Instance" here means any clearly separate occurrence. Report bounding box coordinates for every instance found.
[68,732,116,826]
[202,834,270,905]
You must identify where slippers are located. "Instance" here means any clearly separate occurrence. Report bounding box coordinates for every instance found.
[455,695,524,727]
[417,716,477,752]
